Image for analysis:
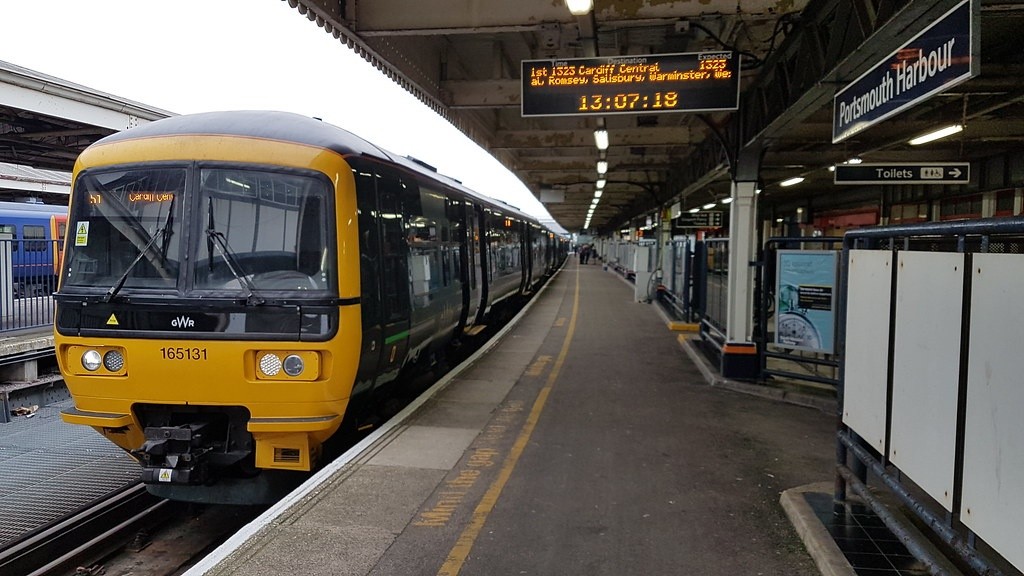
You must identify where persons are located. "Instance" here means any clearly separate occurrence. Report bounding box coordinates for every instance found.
[575,244,597,266]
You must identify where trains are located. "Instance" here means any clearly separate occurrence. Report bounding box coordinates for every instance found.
[51,110,568,503]
[0,197,69,299]
